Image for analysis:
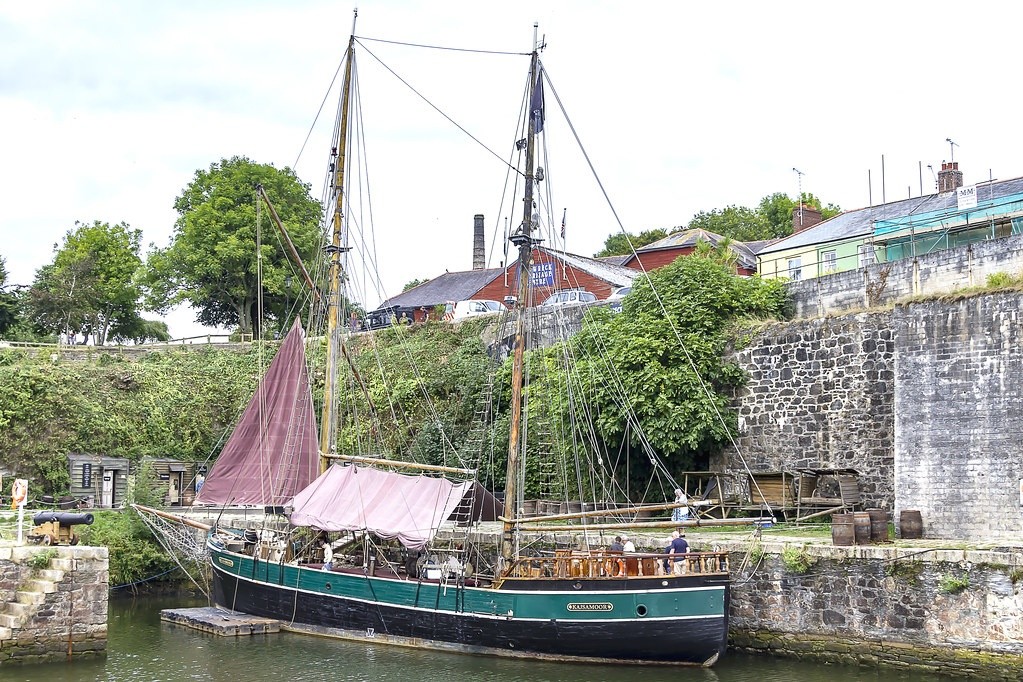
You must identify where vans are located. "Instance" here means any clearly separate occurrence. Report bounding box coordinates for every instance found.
[357,305,415,331]
[455,299,509,320]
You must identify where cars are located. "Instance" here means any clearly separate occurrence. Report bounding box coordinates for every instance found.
[606,285,632,300]
[536,291,598,308]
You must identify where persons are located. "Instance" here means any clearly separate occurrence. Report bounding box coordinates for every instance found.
[195,473,204,494]
[350,312,358,332]
[663,531,690,575]
[68,334,75,345]
[417,552,459,580]
[398,307,429,326]
[610,536,636,554]
[321,540,332,571]
[671,489,688,537]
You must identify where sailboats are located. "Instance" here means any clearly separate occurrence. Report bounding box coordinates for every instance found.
[130,8,778,667]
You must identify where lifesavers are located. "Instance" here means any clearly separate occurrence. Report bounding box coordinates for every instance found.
[605,557,625,577]
[12,480,27,502]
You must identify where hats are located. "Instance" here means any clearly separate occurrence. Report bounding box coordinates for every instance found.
[622,536,630,541]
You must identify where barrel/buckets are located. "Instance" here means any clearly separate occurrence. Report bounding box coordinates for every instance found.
[900,510,922,539]
[521,499,652,525]
[832,514,855,546]
[848,512,871,545]
[864,508,888,541]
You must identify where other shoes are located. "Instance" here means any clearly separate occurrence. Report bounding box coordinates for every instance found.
[679,533,685,538]
